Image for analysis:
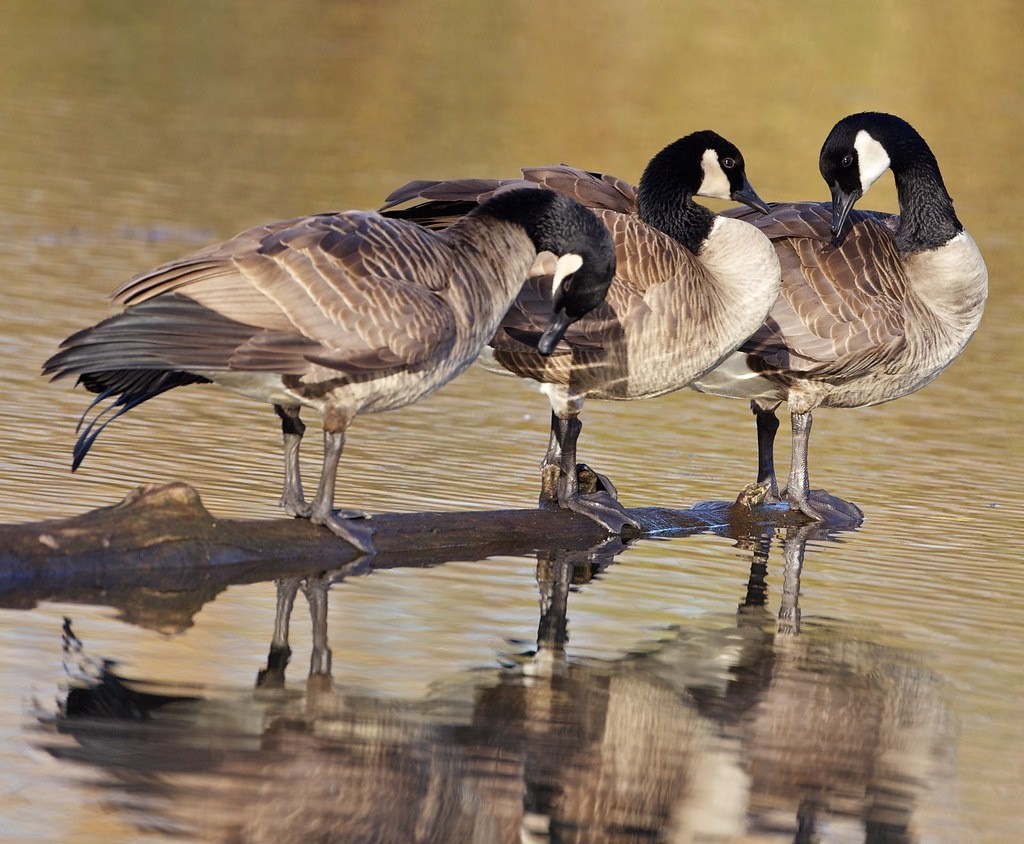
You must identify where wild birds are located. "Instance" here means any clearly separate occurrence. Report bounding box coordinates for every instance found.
[39,106,995,559]
